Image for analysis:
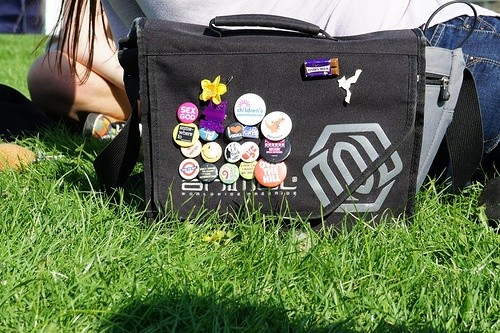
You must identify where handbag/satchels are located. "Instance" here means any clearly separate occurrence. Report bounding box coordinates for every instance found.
[416,45,481,197]
[93,14,426,236]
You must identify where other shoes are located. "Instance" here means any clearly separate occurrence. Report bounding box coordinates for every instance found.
[1,144,36,170]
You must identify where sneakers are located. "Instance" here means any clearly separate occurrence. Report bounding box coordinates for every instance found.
[83,113,143,139]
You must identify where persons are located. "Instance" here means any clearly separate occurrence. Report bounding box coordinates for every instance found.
[101,0,499,231]
[27,0,142,142]
